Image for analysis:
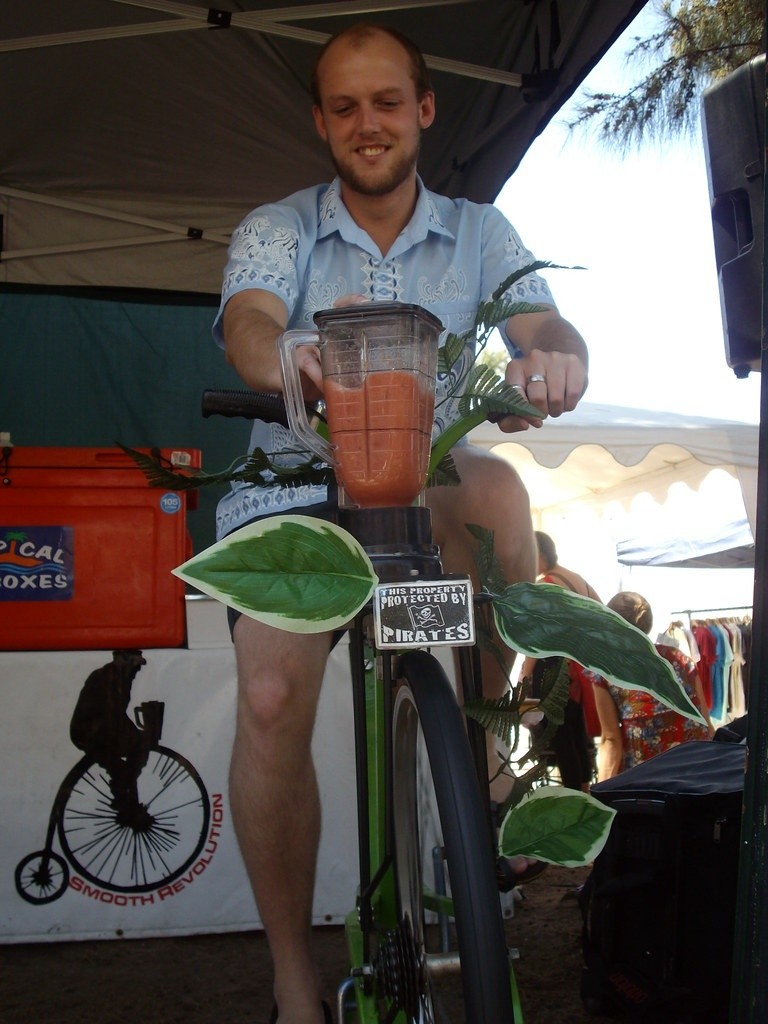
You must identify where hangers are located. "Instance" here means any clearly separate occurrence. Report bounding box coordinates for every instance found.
[663,604,751,635]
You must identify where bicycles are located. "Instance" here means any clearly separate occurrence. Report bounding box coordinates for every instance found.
[200,386,524,1024]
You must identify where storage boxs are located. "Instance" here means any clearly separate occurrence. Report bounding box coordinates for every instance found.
[0,444,203,650]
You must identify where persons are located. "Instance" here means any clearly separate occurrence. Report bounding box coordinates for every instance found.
[520,530,605,792]
[595,591,717,784]
[214,21,586,1024]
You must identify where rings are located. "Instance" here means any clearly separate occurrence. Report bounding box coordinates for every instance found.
[525,375,547,385]
[508,383,524,390]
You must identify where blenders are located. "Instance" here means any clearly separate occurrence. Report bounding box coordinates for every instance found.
[278,302,447,585]
